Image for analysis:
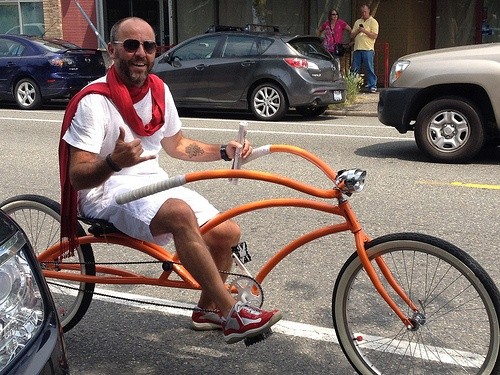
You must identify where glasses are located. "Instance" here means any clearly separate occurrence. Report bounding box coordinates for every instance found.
[112,38,156,55]
[332,13,339,16]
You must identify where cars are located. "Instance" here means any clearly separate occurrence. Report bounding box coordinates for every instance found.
[149,30,347,123]
[376,40,499,164]
[0,33,107,111]
[5,22,58,37]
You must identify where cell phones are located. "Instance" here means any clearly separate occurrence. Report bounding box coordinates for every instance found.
[359,24,364,28]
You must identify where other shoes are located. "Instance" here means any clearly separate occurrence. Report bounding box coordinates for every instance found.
[369,86,377,93]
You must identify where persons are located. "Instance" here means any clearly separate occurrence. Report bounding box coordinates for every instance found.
[317,7,351,73]
[67,16,283,345]
[349,4,378,93]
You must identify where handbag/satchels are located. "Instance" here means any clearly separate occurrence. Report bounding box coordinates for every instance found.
[334,43,344,57]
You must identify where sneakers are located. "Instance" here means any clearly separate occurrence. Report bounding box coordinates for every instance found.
[189,305,226,331]
[217,300,283,344]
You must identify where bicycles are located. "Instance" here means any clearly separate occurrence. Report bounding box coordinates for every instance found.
[0,142,500,375]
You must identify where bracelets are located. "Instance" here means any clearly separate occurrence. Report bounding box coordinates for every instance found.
[106,154,122,172]
[219,143,233,162]
